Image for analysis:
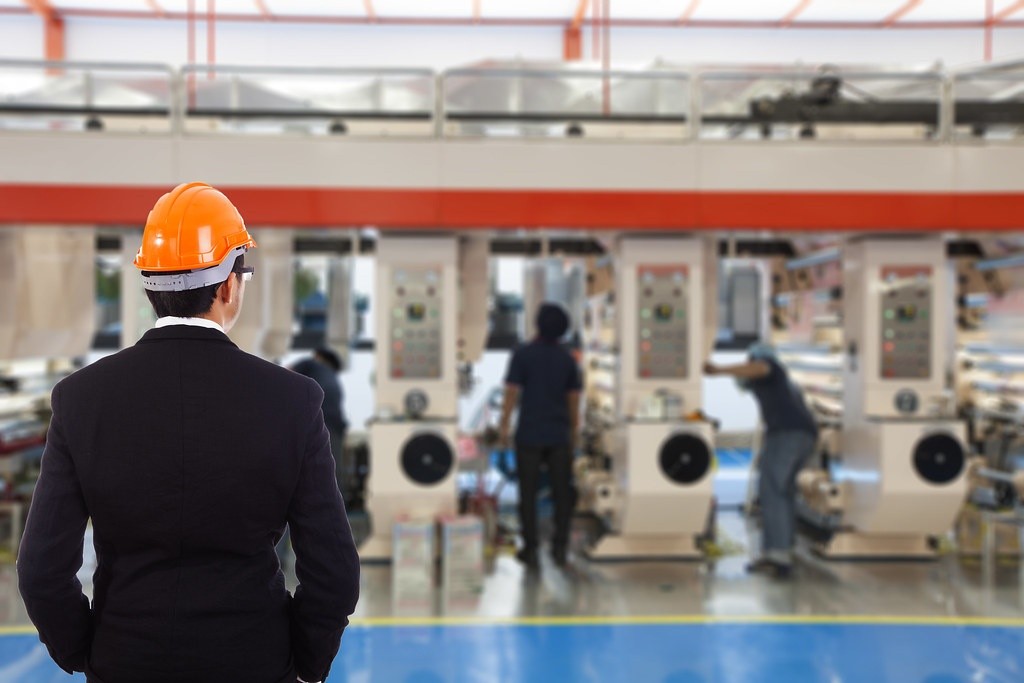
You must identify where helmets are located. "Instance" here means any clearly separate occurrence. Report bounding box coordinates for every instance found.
[135,181,256,293]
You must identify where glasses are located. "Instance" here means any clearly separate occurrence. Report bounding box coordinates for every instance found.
[240,266,254,282]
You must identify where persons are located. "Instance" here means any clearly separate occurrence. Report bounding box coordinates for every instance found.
[703,335,821,578]
[15,180,361,683]
[279,342,354,495]
[496,301,585,570]
